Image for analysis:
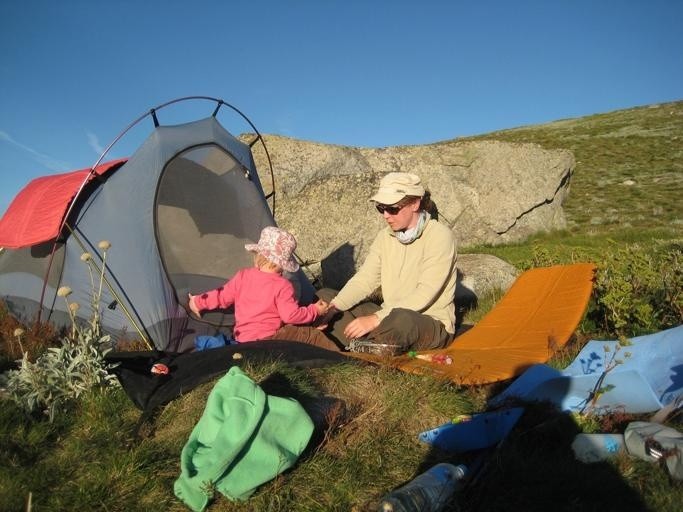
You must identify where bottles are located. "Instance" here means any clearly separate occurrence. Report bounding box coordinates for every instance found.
[377,463,468,512]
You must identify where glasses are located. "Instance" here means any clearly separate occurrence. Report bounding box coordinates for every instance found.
[375,201,414,215]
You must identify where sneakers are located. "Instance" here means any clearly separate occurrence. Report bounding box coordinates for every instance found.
[345,339,402,357]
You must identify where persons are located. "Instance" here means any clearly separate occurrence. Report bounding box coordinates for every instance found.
[187,226,341,352]
[311,171,459,353]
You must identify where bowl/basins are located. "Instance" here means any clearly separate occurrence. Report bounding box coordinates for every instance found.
[353,343,402,357]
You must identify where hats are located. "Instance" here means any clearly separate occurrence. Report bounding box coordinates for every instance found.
[368,173,426,206]
[245,226,300,273]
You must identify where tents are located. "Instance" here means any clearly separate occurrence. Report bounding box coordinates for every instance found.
[1,116,319,354]
